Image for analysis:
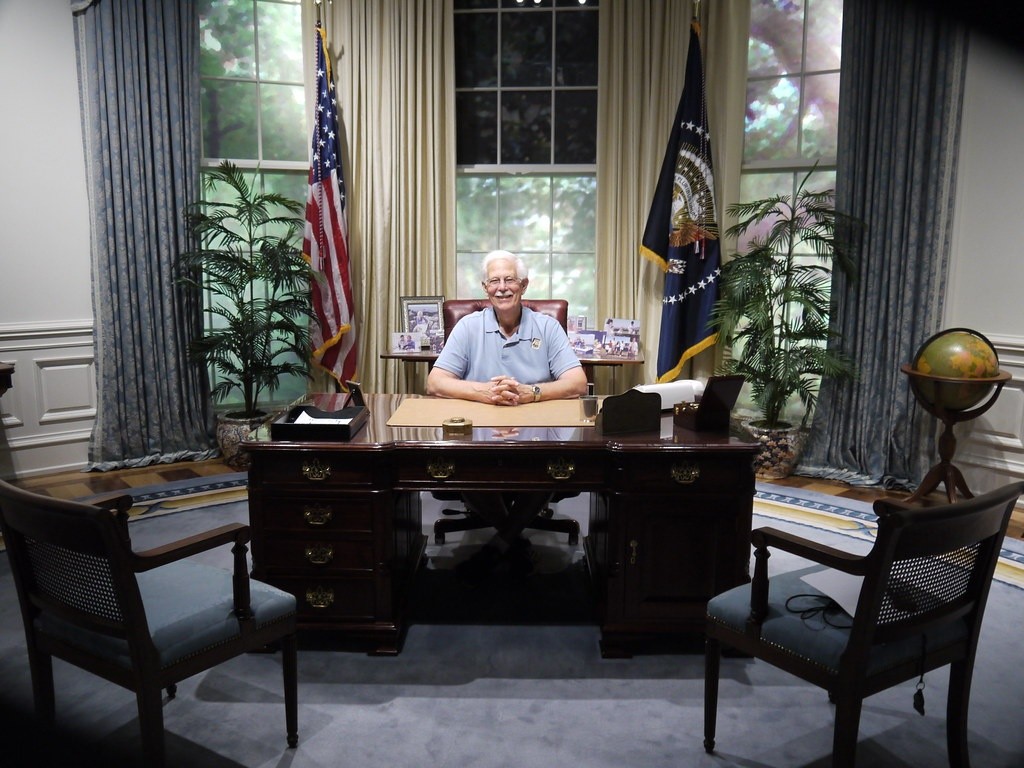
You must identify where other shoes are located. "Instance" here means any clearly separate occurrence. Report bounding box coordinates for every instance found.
[513,537,535,561]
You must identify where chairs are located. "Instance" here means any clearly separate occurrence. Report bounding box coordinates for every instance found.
[428,296,580,559]
[697,477,1024,768]
[0,472,307,768]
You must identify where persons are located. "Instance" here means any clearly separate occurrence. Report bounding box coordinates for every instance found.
[399,335,415,350]
[427,250,588,588]
[416,311,428,331]
[569,319,638,360]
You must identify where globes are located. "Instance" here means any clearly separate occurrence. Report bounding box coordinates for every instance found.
[900,328,1012,505]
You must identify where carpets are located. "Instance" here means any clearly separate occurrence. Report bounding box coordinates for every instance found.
[0,466,1024,768]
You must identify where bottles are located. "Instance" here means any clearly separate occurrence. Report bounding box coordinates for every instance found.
[583,384,597,418]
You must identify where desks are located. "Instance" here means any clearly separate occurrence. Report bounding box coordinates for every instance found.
[0,362,16,398]
[236,387,773,660]
[380,344,646,394]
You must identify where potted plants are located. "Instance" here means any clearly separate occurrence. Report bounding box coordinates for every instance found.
[171,156,330,469]
[701,153,873,483]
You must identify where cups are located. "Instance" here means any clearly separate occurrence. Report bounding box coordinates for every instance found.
[578,397,598,422]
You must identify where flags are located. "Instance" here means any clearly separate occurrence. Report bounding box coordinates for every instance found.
[301,24,361,392]
[639,16,721,385]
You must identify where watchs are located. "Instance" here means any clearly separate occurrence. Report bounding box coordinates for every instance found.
[531,383,540,402]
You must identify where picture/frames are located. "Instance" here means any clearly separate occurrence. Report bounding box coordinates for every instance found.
[398,294,446,342]
